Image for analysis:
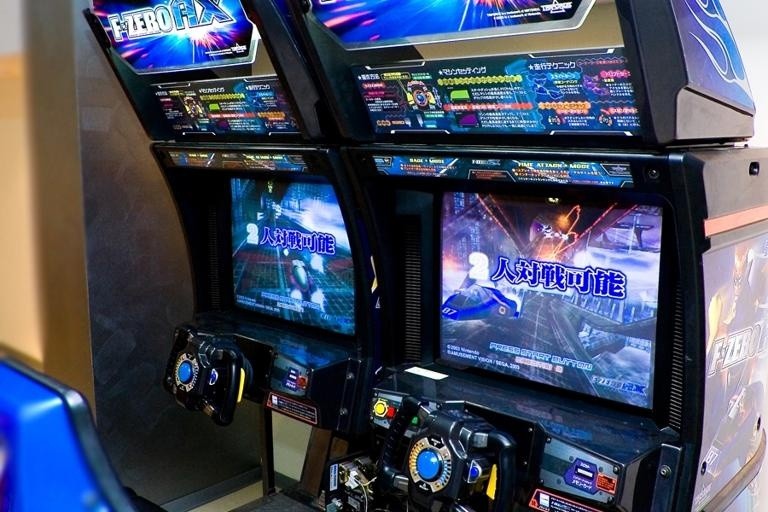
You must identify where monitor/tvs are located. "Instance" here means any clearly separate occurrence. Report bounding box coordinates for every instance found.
[219,172,358,344]
[437,178,666,421]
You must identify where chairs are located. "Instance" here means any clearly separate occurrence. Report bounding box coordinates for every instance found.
[0,354,138,512]
